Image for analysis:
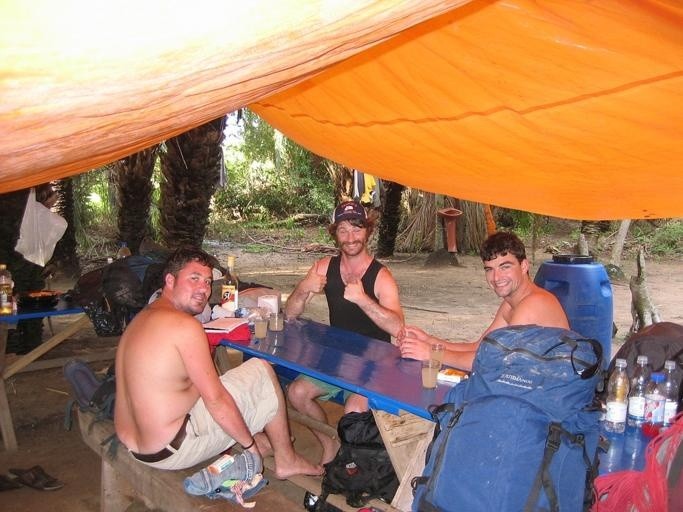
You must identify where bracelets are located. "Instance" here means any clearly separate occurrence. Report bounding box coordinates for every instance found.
[239,439,255,451]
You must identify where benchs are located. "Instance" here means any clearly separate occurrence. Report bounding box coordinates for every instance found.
[0,307,92,452]
[271,364,401,418]
[0,335,124,377]
[73,408,406,512]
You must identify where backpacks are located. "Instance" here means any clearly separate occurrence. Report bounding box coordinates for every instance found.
[319,412,400,508]
[64,359,114,432]
[411,325,610,512]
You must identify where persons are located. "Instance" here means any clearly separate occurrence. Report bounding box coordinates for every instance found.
[395,231,569,374]
[112,247,324,480]
[284,201,405,466]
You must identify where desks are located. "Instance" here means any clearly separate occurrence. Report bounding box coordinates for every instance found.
[594,406,673,477]
[220,314,469,424]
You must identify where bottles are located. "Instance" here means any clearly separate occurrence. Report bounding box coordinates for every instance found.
[602,355,682,437]
[220,254,239,313]
[0,265,13,314]
[116,240,131,260]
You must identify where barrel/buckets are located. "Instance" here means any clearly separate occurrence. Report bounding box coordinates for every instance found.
[533,251,615,371]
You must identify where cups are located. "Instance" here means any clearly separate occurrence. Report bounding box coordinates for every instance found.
[253,313,284,339]
[420,342,444,389]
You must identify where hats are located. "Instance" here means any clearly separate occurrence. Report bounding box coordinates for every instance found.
[333,201,367,222]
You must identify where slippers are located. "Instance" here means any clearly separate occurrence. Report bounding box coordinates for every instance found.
[0,465,63,491]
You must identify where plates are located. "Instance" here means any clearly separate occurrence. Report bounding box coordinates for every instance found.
[16,290,59,312]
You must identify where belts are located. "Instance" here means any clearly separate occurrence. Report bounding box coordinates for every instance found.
[131,413,190,462]
